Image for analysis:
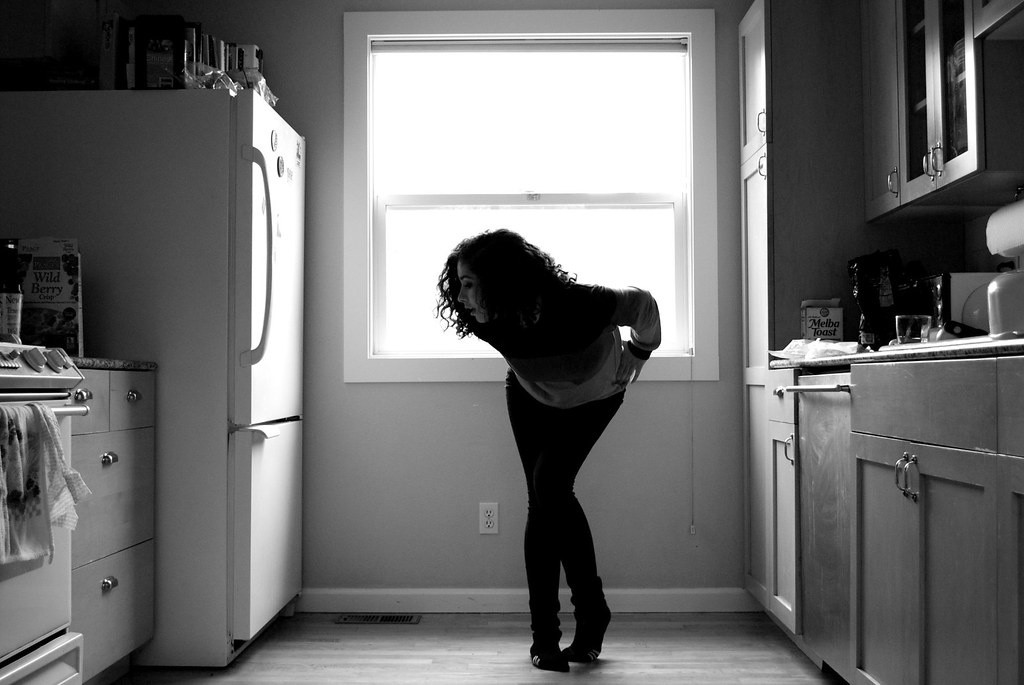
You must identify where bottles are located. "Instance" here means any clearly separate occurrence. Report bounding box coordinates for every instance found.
[1,246,25,342]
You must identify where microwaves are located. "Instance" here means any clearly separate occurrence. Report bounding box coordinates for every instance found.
[895,271,1000,339]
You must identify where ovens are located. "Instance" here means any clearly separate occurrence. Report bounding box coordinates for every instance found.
[0,388,93,665]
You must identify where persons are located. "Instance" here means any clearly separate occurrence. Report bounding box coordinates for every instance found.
[436,227,661,671]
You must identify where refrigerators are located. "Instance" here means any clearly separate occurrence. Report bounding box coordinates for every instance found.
[1,89,309,669]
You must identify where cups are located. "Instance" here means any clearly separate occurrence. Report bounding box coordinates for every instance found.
[894,313,931,345]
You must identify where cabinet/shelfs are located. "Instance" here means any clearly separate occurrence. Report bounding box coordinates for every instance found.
[846,346,997,685]
[860,0,988,228]
[739,0,799,655]
[0,86,307,669]
[71,345,163,679]
